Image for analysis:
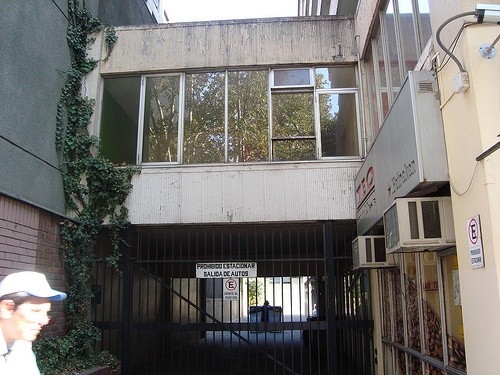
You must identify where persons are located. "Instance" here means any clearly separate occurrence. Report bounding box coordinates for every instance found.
[0,271,69,374]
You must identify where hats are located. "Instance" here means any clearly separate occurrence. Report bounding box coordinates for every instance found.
[0,271,67,301]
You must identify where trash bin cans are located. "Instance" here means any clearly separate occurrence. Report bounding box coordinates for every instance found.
[250,306,282,332]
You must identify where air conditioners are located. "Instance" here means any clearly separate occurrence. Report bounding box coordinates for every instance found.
[351,235,399,271]
[382,196,456,254]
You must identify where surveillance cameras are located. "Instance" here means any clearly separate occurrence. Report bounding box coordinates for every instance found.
[475,3,500,26]
